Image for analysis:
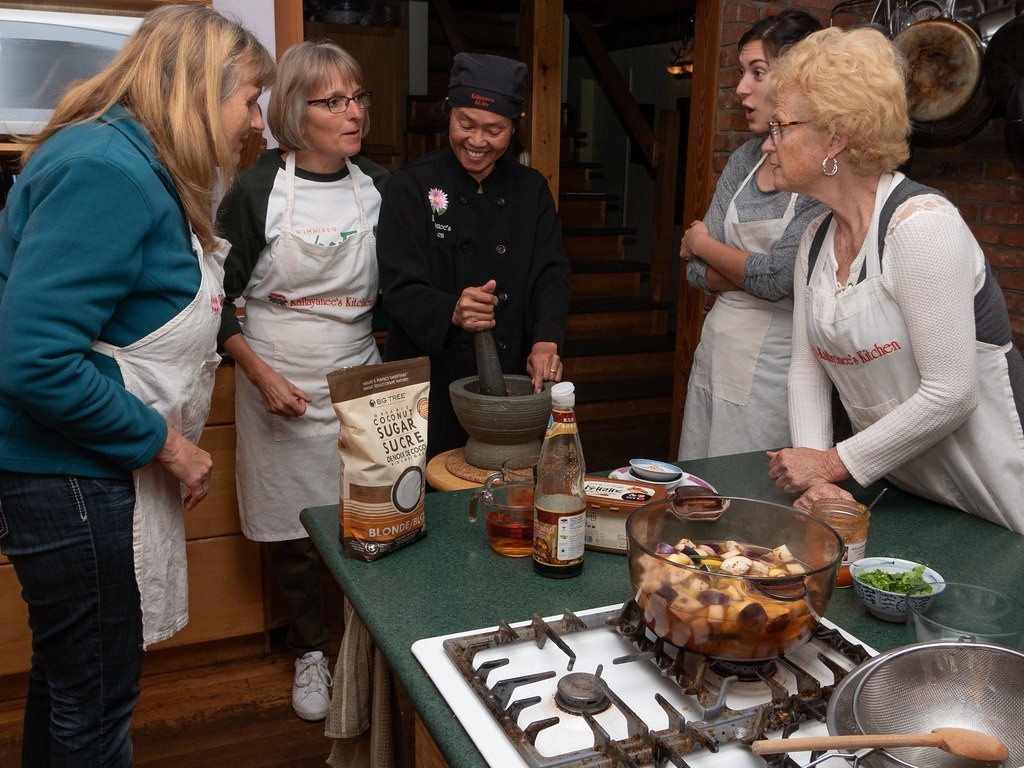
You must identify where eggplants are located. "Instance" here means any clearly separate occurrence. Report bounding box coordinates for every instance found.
[653,540,776,634]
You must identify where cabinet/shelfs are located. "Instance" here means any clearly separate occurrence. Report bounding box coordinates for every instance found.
[303,21,406,173]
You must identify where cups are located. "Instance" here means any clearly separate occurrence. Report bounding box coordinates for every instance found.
[469,484,536,557]
[484,455,540,518]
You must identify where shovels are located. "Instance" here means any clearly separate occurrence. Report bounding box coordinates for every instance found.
[751,728,1009,761]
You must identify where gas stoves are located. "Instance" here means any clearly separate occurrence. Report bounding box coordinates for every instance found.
[410,603,1024,768]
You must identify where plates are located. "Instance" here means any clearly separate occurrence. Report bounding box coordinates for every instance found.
[629,458,683,490]
[608,466,718,511]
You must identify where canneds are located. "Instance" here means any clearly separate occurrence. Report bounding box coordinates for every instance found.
[808,497,871,588]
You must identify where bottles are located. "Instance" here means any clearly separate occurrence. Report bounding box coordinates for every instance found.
[805,498,870,588]
[534,382,586,579]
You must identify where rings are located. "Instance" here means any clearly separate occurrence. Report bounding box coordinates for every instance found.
[550,369,557,373]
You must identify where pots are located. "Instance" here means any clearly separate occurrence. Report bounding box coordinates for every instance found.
[625,485,844,661]
[867,0,1024,162]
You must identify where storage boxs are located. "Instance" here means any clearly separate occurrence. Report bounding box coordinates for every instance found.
[584,475,669,556]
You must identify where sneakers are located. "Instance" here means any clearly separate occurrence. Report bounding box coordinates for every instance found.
[289,650,334,721]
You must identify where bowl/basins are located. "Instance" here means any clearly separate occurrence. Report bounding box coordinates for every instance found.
[826,638,1024,768]
[907,582,1024,656]
[849,557,946,622]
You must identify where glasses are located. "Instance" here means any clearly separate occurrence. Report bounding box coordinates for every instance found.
[769,120,815,145]
[307,92,373,114]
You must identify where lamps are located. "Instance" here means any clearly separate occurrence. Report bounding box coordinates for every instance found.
[664,16,693,81]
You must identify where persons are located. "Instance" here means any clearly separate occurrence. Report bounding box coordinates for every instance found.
[676,9,832,461]
[214,38,403,721]
[758,26,1024,535]
[0,3,277,768]
[372,51,571,493]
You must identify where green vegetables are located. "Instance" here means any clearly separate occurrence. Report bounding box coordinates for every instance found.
[860,565,931,595]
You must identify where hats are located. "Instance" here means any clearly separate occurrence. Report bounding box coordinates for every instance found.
[443,52,529,118]
